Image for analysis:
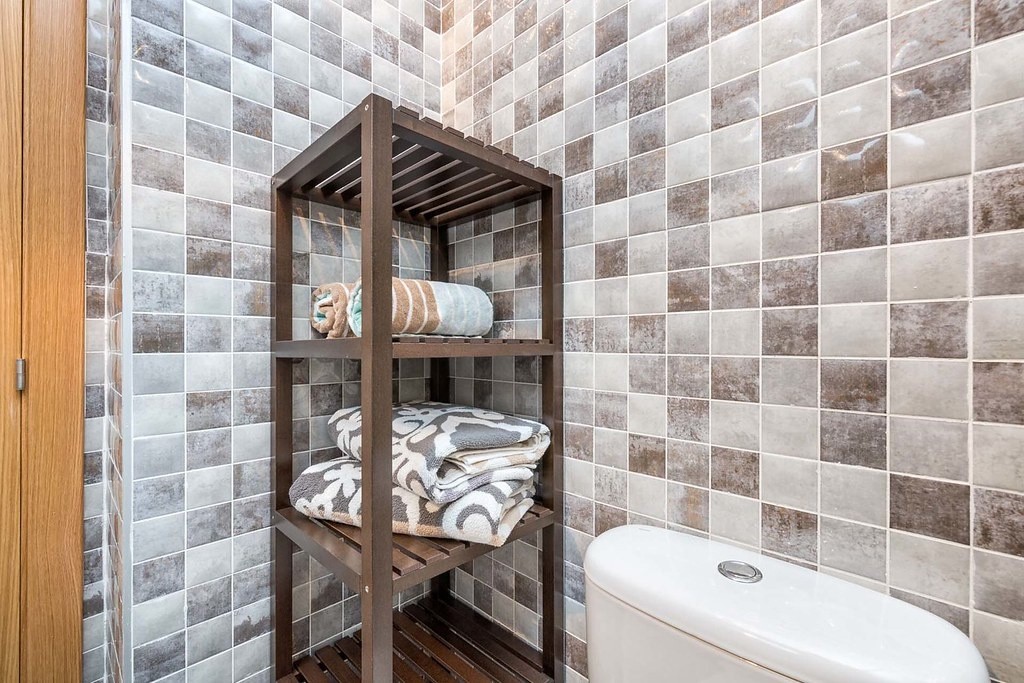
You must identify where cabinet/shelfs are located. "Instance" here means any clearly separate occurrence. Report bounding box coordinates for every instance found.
[268,93,565,683]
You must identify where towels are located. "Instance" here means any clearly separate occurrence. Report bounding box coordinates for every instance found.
[310,275,494,341]
[290,398,549,548]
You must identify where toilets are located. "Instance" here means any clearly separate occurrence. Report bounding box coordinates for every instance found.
[584,523,991,682]
